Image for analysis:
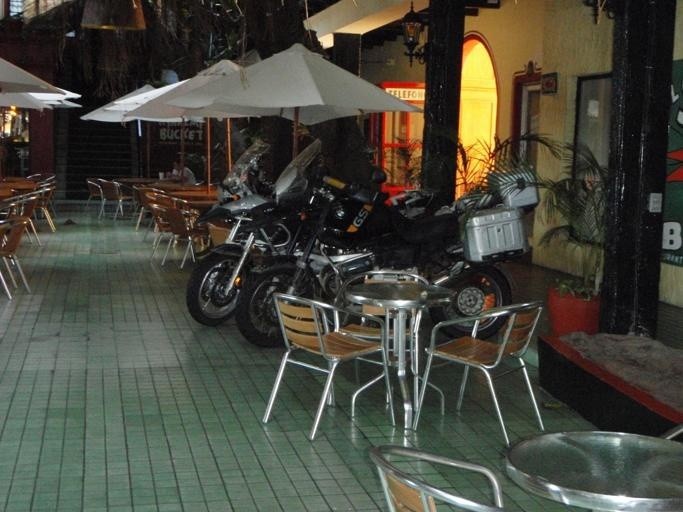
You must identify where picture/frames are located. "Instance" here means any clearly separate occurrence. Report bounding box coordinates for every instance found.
[568,72,612,248]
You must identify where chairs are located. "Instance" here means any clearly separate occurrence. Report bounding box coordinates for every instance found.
[325,270,448,429]
[367,443,504,512]
[259,291,397,442]
[410,295,545,449]
[83,175,211,271]
[0,171,58,299]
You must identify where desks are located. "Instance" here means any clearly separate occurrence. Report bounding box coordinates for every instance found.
[504,431,683,512]
[341,280,457,450]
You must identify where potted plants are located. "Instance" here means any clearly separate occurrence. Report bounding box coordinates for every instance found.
[462,141,614,337]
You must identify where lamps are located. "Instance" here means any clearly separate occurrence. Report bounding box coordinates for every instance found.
[583,0,626,24]
[396,2,432,67]
